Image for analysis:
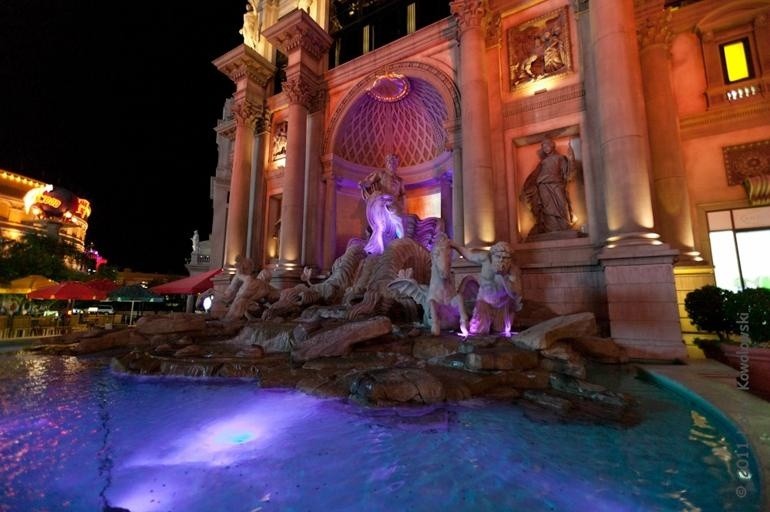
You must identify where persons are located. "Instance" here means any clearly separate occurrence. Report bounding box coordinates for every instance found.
[222,267,280,319]
[355,151,409,216]
[440,234,524,335]
[520,137,578,233]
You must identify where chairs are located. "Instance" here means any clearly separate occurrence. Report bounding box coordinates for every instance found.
[0,313,124,339]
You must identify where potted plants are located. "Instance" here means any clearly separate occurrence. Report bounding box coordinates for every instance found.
[685,285,770,394]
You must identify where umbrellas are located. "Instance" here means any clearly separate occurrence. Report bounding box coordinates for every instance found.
[0,271,166,327]
